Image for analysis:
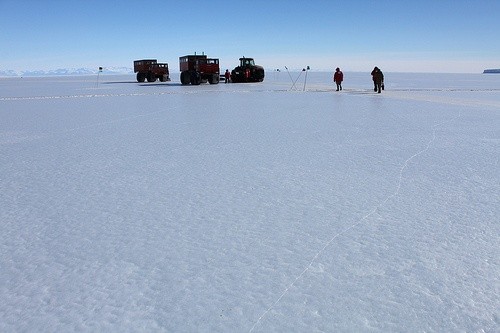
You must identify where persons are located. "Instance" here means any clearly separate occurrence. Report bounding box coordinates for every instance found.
[334,68,344,91]
[224,69,230,83]
[371,67,383,93]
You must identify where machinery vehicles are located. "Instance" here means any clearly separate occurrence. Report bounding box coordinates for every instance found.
[133,58,168,82]
[179,55,219,85]
[232,56,264,82]
